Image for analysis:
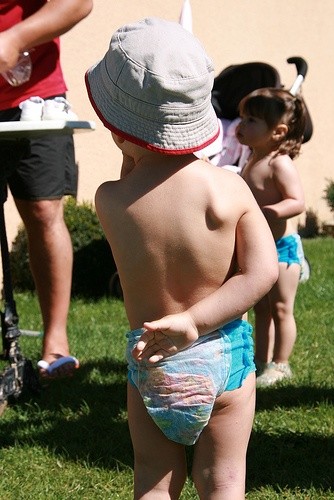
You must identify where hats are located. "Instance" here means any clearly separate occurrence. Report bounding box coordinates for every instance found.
[84,16,220,155]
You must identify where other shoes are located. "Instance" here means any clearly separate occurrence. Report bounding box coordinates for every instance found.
[255,362,291,387]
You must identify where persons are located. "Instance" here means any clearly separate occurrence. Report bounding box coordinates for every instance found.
[0,0,94,386]
[85,19,279,500]
[235,89,305,388]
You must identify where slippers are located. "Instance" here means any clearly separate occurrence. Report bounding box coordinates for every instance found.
[37,355,79,386]
